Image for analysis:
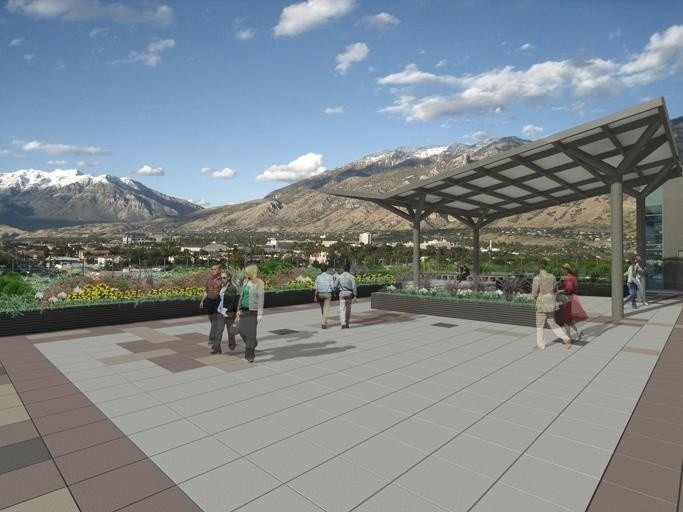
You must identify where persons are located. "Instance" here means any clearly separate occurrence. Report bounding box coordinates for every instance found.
[314,265,336,330]
[623,256,649,310]
[529,257,572,351]
[334,264,358,329]
[208,271,237,354]
[199,264,223,347]
[557,262,588,343]
[235,264,264,363]
[462,265,470,281]
